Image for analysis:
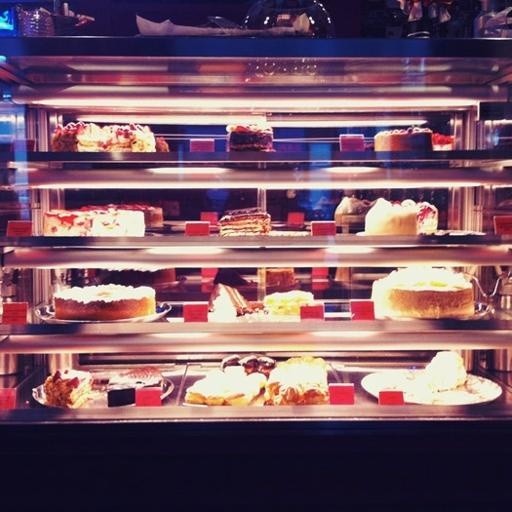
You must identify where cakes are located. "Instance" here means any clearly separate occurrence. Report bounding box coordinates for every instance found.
[81,204,164,230]
[373,126,456,152]
[365,198,439,237]
[154,138,171,151]
[43,351,468,412]
[51,122,156,152]
[42,209,146,238]
[54,266,476,322]
[333,196,398,226]
[227,124,274,152]
[218,213,271,238]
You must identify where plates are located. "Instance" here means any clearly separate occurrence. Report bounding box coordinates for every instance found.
[34,300,172,323]
[361,367,503,405]
[374,300,491,319]
[31,377,175,408]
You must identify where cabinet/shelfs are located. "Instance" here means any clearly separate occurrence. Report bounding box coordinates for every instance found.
[1,33,512,435]
